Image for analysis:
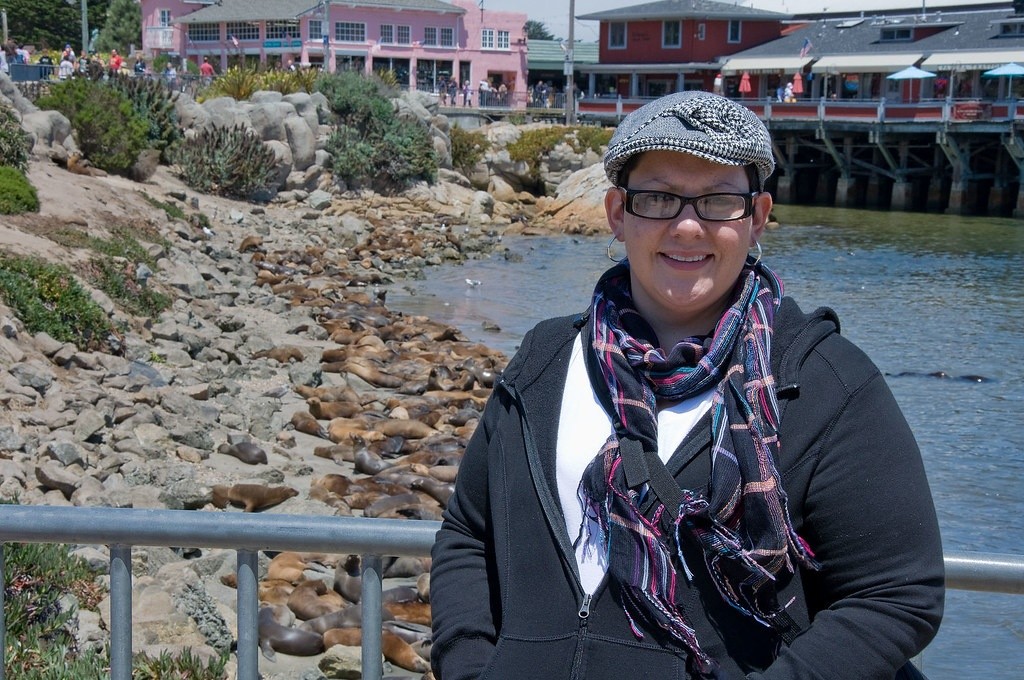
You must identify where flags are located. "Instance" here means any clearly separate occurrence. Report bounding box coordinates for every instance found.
[799,36,812,59]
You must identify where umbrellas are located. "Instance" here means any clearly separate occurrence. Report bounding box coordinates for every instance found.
[791,71,803,93]
[983,62,1024,99]
[885,65,937,103]
[738,71,751,103]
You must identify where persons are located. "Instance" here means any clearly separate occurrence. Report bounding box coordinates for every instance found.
[117,61,130,76]
[134,54,146,76]
[479,76,585,109]
[274,60,295,72]
[58,42,106,81]
[438,75,447,105]
[768,82,794,103]
[163,62,176,89]
[1,41,34,73]
[448,76,458,105]
[462,80,474,106]
[38,48,54,80]
[430,91,946,678]
[106,49,122,78]
[199,57,218,88]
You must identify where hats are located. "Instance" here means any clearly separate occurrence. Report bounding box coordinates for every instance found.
[604,90,775,193]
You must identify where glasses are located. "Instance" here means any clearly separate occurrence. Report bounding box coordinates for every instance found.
[616,185,759,222]
